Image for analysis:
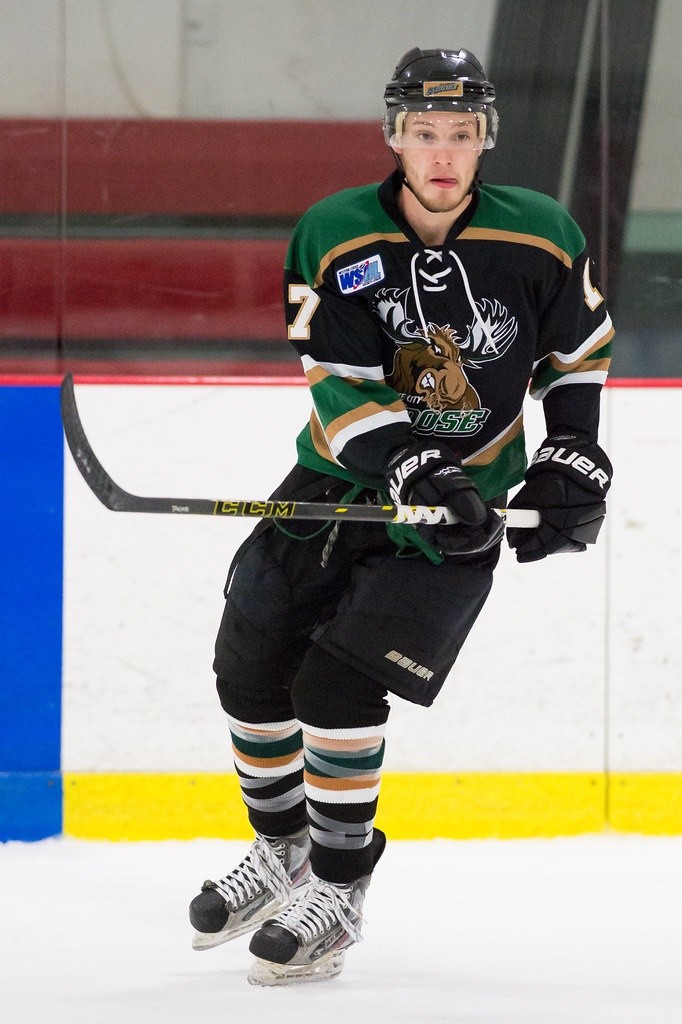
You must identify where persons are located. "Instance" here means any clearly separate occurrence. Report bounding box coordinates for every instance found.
[188,46,618,987]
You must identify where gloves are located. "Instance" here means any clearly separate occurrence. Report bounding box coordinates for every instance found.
[506,429,614,563]
[384,436,504,562]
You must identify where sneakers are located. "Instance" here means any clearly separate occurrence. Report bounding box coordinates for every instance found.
[247,827,386,986]
[189,823,312,952]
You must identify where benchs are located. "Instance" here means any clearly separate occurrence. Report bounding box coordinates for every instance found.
[0,116,401,217]
[0,238,292,344]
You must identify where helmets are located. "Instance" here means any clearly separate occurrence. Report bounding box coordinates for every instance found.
[384,48,496,111]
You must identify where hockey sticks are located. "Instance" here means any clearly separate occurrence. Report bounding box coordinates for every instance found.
[57,374,542,529]
